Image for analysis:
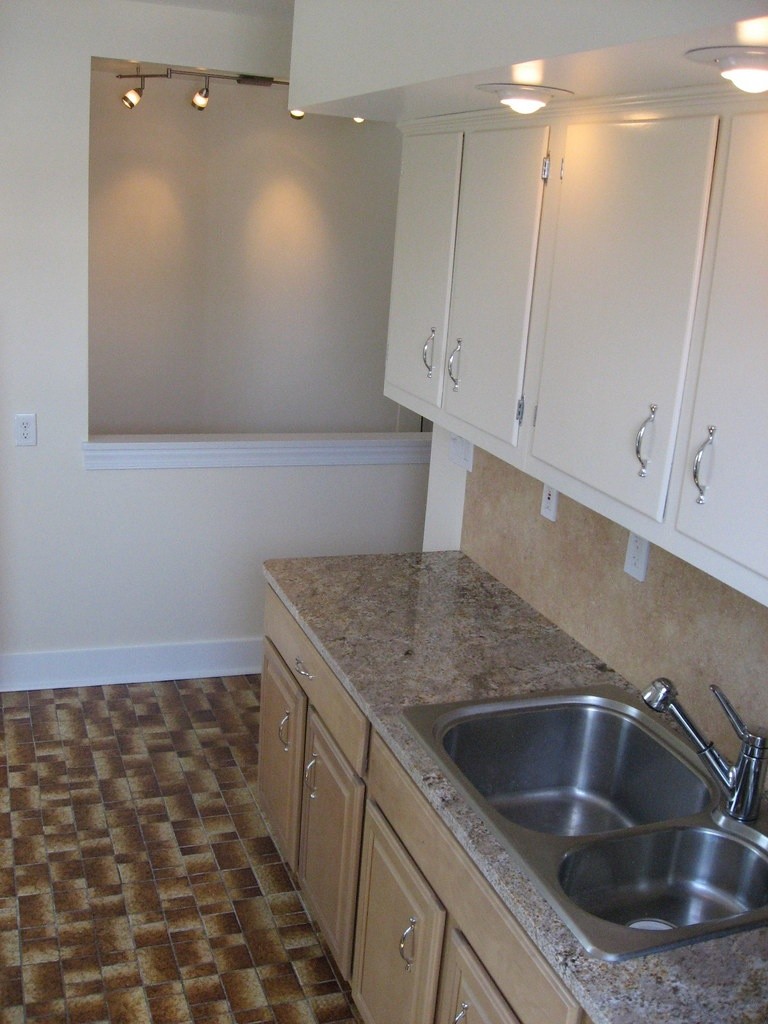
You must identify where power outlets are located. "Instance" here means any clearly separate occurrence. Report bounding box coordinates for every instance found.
[14,414,35,444]
[541,483,558,522]
[623,531,649,581]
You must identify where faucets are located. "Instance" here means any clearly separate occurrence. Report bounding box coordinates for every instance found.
[640,678,768,823]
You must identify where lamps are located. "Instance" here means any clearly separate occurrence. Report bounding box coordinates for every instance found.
[117,66,305,119]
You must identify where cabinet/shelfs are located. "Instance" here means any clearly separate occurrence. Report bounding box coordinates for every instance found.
[383,85,768,608]
[259,583,594,1024]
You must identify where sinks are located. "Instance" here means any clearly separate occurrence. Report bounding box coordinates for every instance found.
[532,808,768,962]
[399,678,732,839]
